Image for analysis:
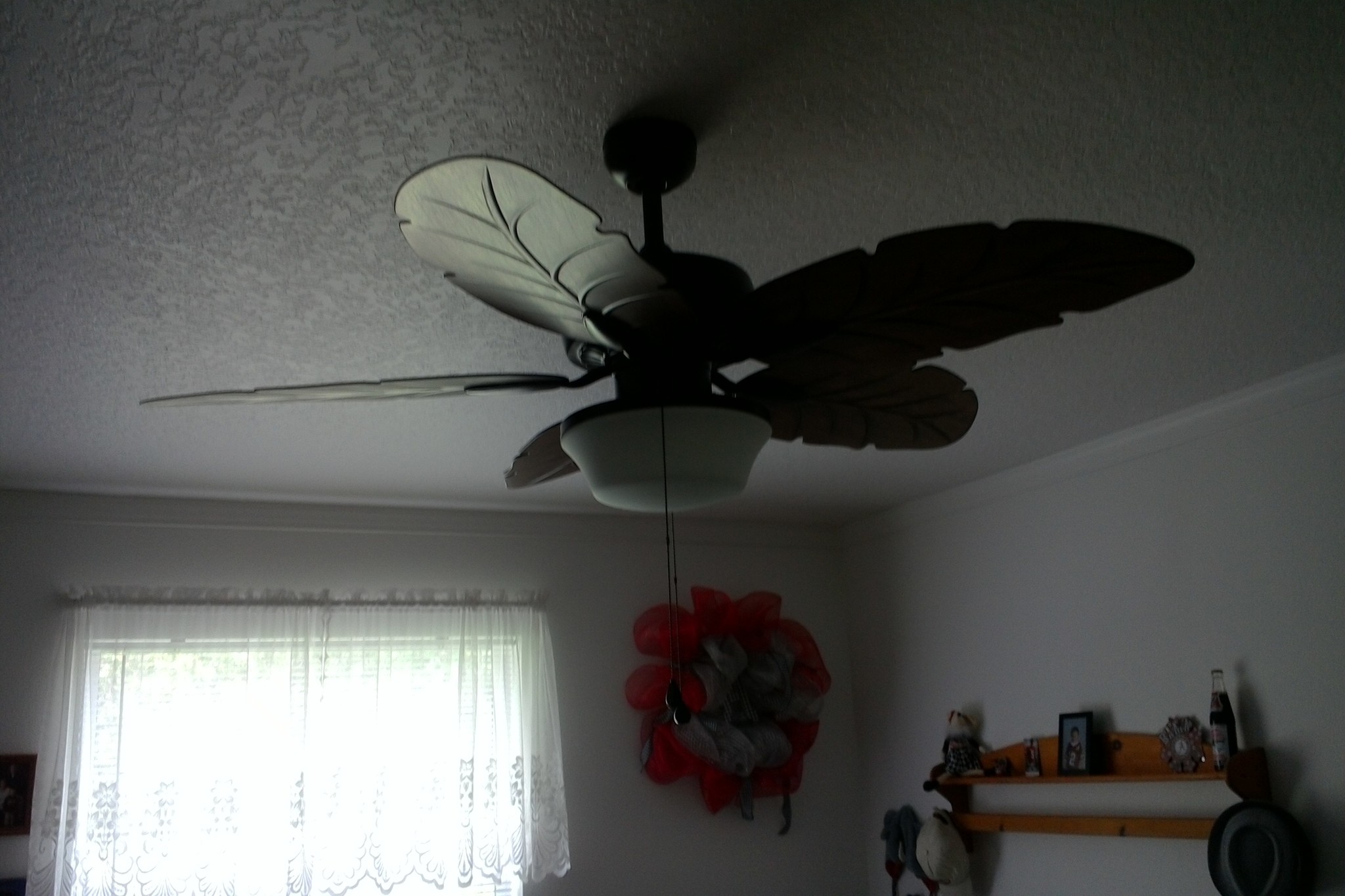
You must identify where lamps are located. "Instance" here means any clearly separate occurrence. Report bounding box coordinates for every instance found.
[560,396,772,513]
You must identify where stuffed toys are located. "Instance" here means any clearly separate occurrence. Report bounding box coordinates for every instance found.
[941,709,984,776]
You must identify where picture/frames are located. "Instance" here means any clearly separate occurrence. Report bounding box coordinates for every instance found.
[1056,710,1091,776]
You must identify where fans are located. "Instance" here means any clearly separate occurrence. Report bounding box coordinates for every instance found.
[139,98,1195,486]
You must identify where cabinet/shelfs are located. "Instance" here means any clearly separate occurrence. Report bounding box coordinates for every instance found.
[922,731,1228,842]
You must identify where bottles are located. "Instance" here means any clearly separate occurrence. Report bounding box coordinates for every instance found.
[1209,670,1239,772]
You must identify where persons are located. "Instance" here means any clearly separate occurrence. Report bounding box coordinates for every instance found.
[1026,738,1036,767]
[1065,727,1082,769]
[0,762,25,828]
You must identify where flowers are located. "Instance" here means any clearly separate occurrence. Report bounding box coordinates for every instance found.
[624,585,833,834]
